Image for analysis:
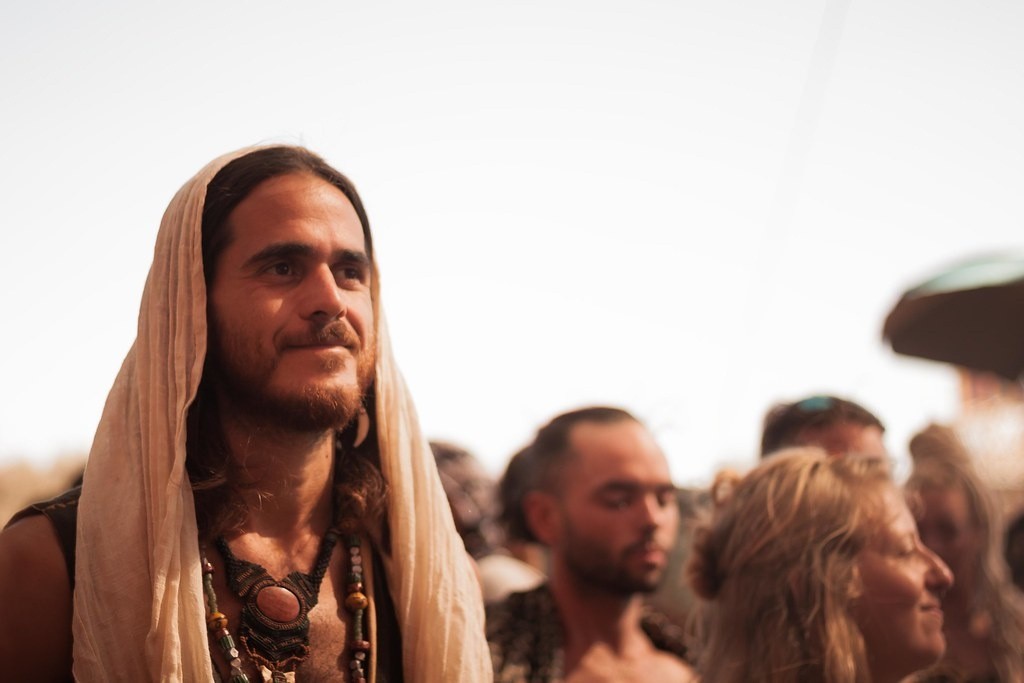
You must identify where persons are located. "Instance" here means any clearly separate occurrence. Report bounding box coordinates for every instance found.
[430,396,1024,683]
[0,144,492,683]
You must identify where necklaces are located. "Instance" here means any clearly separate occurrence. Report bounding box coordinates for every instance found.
[193,514,370,683]
